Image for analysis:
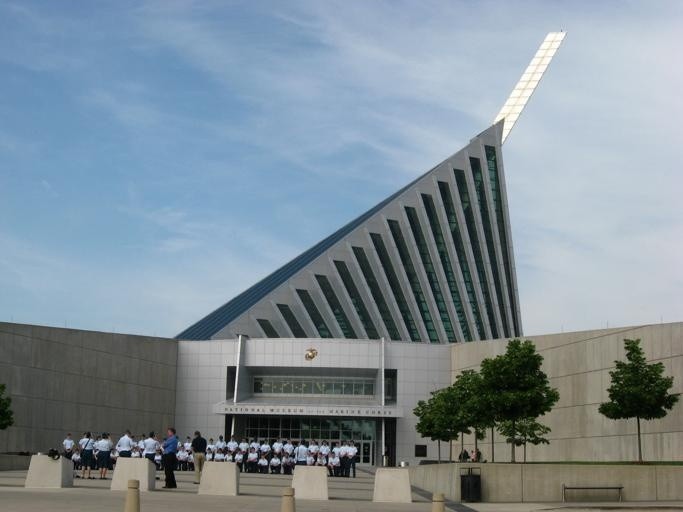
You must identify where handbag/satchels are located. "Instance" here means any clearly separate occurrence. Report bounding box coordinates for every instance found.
[80,451,89,459]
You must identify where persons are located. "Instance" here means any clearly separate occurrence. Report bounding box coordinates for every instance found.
[63,428,357,488]
[459,449,481,462]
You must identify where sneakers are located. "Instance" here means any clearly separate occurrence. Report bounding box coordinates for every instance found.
[82,477,107,479]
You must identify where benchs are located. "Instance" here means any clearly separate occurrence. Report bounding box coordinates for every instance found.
[562,484,624,503]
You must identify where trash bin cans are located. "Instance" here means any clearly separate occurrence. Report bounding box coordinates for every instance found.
[460,466,482,503]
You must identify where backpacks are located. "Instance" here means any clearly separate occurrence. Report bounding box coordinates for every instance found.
[48,447,60,460]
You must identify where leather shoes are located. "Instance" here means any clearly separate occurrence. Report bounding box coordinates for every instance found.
[193,481,201,484]
[162,485,177,488]
